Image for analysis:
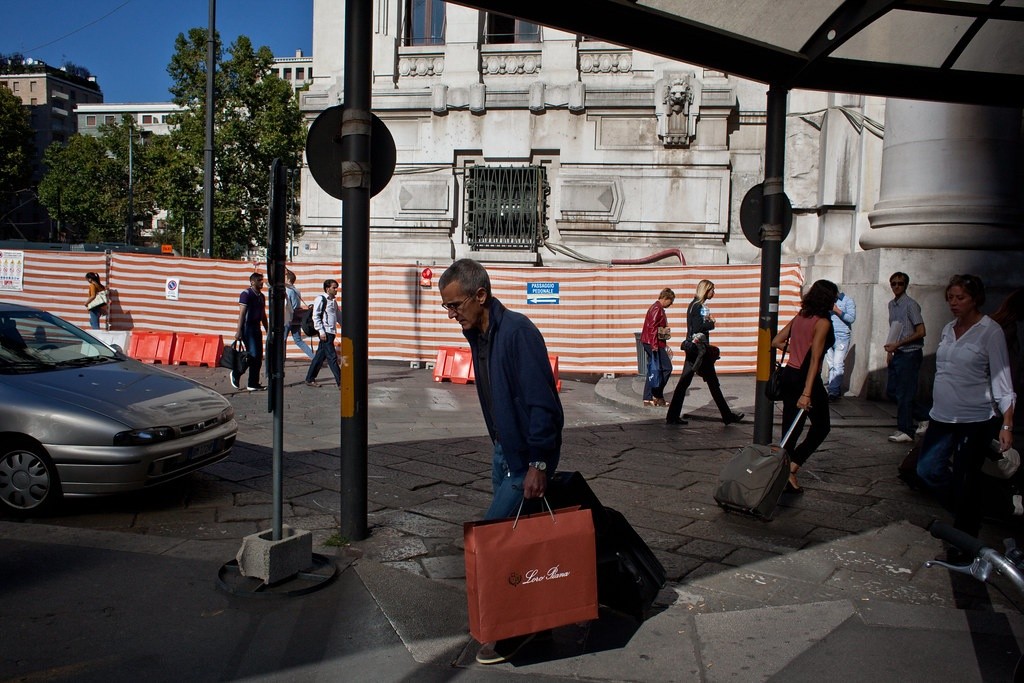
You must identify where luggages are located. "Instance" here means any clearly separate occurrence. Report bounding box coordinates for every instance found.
[713,404,810,522]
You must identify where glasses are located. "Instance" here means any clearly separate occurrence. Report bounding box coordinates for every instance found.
[442,292,478,312]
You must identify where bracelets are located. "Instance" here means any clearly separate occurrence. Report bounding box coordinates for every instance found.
[801,394,810,397]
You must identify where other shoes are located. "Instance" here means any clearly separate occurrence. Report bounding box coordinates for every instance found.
[828,395,836,403]
[654,397,669,407]
[788,480,803,494]
[305,380,321,387]
[644,399,655,407]
[667,418,689,425]
[724,412,744,426]
[475,632,537,664]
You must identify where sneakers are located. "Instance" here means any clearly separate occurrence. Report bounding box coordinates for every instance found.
[916,420,930,434]
[247,383,267,391]
[230,371,239,389]
[888,432,913,443]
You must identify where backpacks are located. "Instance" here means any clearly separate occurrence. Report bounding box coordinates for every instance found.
[302,295,327,337]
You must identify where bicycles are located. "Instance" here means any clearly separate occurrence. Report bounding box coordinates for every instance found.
[922,521,1024,610]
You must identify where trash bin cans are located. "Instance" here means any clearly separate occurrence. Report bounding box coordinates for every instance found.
[634,332,648,377]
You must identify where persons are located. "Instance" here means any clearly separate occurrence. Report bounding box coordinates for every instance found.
[640,288,675,406]
[665,280,745,426]
[230,273,268,391]
[439,259,564,665]
[917,274,1024,562]
[84,272,106,329]
[827,292,856,399]
[282,270,328,368]
[772,280,838,492]
[305,279,341,390]
[884,272,930,441]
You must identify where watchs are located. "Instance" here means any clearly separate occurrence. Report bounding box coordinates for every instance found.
[1001,425,1013,432]
[529,461,547,470]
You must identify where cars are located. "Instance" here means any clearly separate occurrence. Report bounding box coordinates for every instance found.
[0,301,240,519]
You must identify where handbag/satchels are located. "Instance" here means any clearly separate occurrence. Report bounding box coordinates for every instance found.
[647,348,661,389]
[294,308,309,322]
[220,339,250,374]
[88,285,107,311]
[464,485,598,644]
[764,361,787,400]
